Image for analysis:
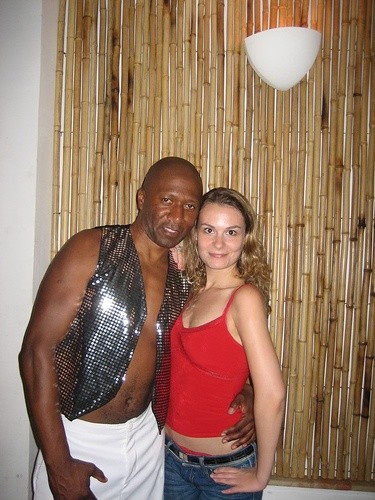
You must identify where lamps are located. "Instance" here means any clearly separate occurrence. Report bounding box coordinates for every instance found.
[244,27,321,91]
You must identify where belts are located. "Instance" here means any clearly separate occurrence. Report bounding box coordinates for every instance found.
[165,436,257,465]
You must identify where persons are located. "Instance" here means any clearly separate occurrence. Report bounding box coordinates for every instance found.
[163,187,287,500]
[18,156,257,500]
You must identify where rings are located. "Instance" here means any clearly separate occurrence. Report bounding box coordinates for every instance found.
[177,246,183,253]
